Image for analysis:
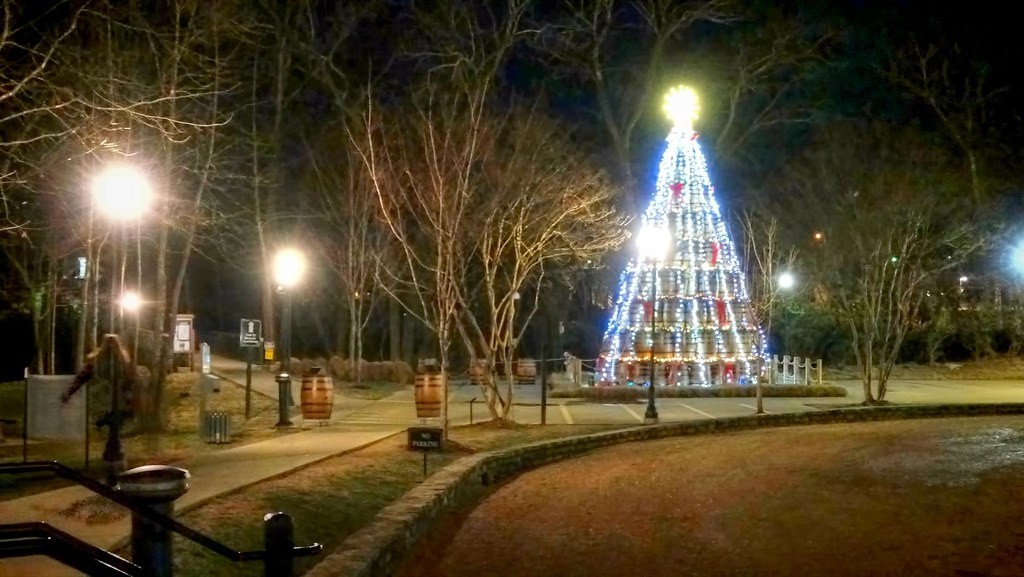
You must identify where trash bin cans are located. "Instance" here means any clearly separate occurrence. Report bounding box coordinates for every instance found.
[199,408,230,444]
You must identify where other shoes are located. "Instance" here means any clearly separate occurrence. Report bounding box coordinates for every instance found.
[104,453,124,463]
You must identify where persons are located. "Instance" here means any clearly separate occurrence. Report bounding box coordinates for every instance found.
[61,334,138,462]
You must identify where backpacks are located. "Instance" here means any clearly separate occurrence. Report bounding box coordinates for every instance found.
[87,357,127,424]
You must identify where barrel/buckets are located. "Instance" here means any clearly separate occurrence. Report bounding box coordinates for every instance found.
[469,358,489,385]
[300,374,334,420]
[414,371,443,417]
[517,358,536,384]
